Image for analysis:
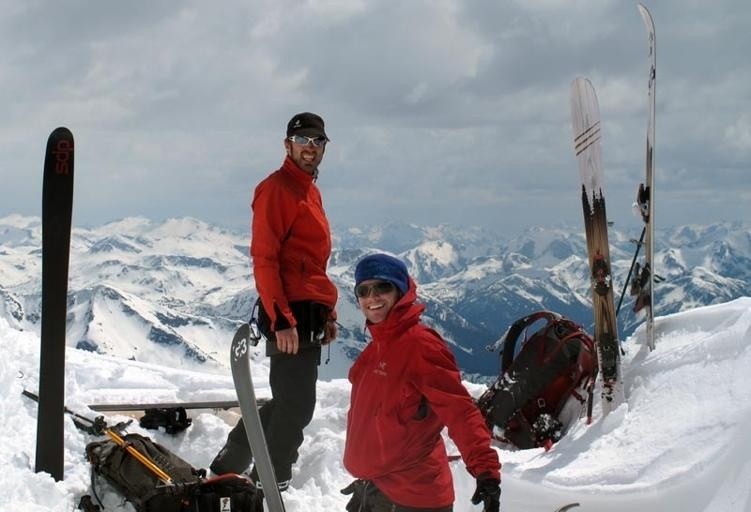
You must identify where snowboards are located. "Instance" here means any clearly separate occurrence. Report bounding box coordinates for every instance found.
[568,77,623,417]
[635,4,656,352]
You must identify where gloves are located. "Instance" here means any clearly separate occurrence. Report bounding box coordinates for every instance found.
[472,479,501,511]
[340,479,368,512]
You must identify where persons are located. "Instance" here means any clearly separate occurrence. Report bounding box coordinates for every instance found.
[209,111,337,502]
[339,249,505,512]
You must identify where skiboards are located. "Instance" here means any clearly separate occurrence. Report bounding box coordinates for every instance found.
[87,399,271,412]
[230,323,285,512]
[34,127,74,483]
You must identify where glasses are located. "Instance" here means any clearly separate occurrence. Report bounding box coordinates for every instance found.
[355,282,397,297]
[288,135,326,146]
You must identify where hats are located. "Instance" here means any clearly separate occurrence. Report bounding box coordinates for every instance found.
[287,113,330,143]
[355,253,410,296]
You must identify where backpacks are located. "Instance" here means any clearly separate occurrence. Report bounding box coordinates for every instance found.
[86,433,257,512]
[479,310,600,450]
